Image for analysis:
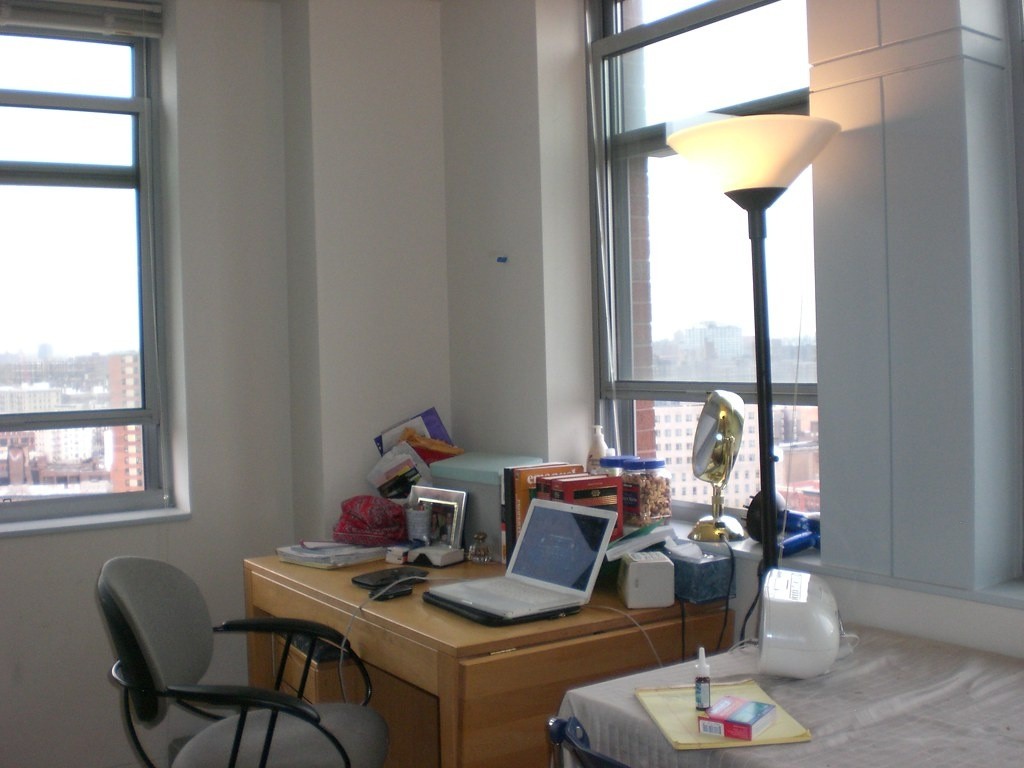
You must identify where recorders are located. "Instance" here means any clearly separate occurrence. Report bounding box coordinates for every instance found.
[760,322,838,679]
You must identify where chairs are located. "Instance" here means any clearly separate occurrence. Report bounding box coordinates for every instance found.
[96,557,388,768]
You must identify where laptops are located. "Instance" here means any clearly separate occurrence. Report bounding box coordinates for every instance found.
[429,498,619,620]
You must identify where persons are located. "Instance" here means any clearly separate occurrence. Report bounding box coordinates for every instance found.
[419,501,453,545]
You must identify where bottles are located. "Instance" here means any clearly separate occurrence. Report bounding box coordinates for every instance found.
[694,647,711,710]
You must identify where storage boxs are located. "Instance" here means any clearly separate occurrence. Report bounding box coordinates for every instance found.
[429,450,543,564]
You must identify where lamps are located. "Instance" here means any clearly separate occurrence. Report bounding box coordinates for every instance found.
[666,114,842,592]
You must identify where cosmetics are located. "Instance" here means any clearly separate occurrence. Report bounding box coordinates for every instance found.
[586,423,607,472]
[467,530,493,563]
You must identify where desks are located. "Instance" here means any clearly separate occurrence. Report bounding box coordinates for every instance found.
[243,535,736,768]
[559,624,1024,768]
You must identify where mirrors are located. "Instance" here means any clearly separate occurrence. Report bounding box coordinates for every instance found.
[688,390,746,543]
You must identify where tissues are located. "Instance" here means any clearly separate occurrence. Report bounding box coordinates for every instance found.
[662,535,733,604]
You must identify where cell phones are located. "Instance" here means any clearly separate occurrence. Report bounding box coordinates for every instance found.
[369,583,413,601]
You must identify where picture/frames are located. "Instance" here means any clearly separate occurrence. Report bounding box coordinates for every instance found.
[410,485,468,549]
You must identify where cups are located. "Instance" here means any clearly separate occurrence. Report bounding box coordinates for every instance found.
[406,509,431,541]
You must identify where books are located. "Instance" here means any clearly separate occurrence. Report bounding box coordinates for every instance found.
[636,675,813,750]
[500,461,624,571]
[276,537,388,568]
[606,518,678,563]
